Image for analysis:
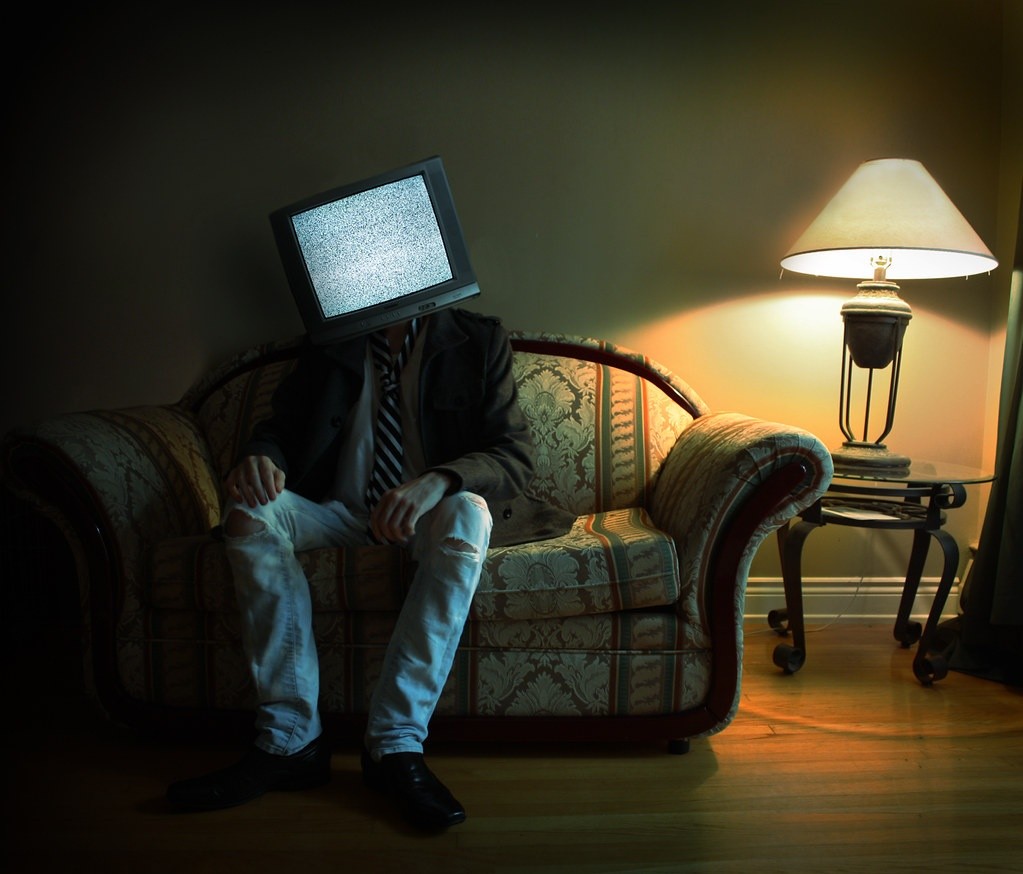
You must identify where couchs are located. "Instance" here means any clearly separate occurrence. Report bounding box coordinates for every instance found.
[36,330,832,761]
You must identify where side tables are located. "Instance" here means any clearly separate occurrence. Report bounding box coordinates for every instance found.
[756,457,998,687]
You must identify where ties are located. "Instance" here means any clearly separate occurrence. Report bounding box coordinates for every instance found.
[364,314,426,547]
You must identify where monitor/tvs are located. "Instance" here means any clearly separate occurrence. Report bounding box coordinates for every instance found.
[269,157,482,345]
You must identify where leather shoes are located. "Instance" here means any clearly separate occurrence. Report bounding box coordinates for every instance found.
[360,747,464,830]
[165,736,331,813]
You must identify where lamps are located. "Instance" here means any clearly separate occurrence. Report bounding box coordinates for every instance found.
[780,158,998,446]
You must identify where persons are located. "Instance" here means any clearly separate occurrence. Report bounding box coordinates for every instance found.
[170,309,536,827]
